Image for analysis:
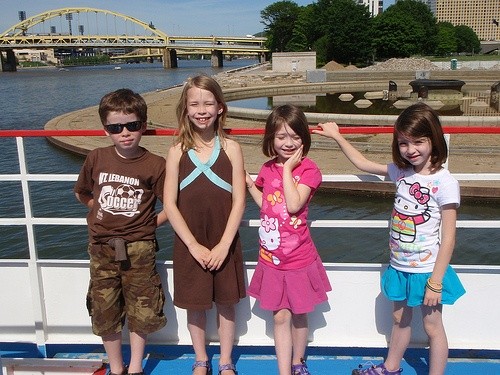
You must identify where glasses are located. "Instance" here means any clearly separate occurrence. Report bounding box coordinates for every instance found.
[104,121,143,134]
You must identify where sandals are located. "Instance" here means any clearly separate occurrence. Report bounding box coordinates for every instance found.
[192,361,211,375]
[217,364,237,375]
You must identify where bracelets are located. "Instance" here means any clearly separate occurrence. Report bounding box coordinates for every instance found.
[427,279,442,294]
[247,183,253,189]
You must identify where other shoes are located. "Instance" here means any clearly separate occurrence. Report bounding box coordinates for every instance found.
[108,366,127,375]
[127,368,144,375]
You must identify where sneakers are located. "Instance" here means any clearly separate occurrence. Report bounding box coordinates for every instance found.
[291,357,311,375]
[352,363,403,375]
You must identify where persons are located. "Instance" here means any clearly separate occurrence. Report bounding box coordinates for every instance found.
[165,75,247,375]
[311,102,466,375]
[74,88,168,375]
[245,103,333,375]
